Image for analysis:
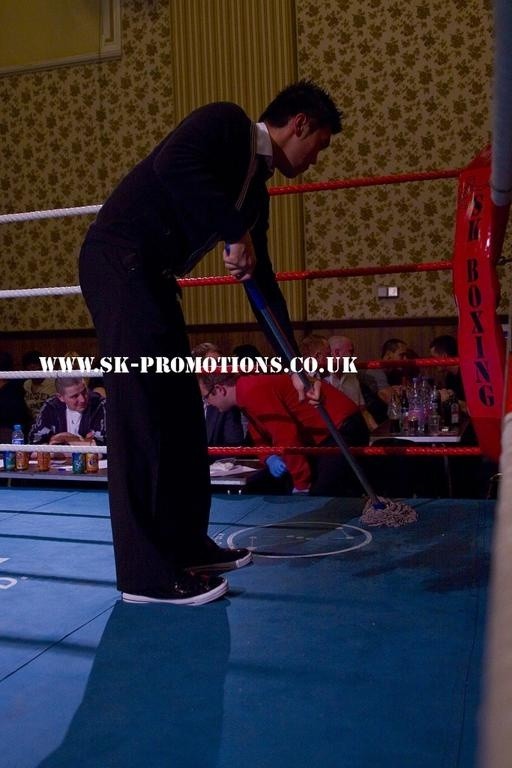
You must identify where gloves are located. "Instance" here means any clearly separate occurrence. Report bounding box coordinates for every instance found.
[265,455,287,480]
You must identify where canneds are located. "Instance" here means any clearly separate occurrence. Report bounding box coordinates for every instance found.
[72,453,85,474]
[3,451,16,471]
[15,451,29,471]
[85,453,98,473]
[37,452,50,472]
[407,416,418,437]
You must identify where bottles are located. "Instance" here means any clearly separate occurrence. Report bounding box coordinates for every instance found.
[12,425,24,444]
[387,377,460,435]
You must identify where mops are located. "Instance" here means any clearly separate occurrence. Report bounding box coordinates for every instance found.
[223,245,418,530]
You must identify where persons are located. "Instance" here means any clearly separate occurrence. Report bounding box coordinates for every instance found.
[73,72,343,609]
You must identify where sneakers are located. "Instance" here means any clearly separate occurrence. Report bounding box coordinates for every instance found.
[121,570,229,607]
[180,542,252,573]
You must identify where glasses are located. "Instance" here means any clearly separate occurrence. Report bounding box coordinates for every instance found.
[203,385,217,404]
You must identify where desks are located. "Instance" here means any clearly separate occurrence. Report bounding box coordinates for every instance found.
[0,451,267,497]
